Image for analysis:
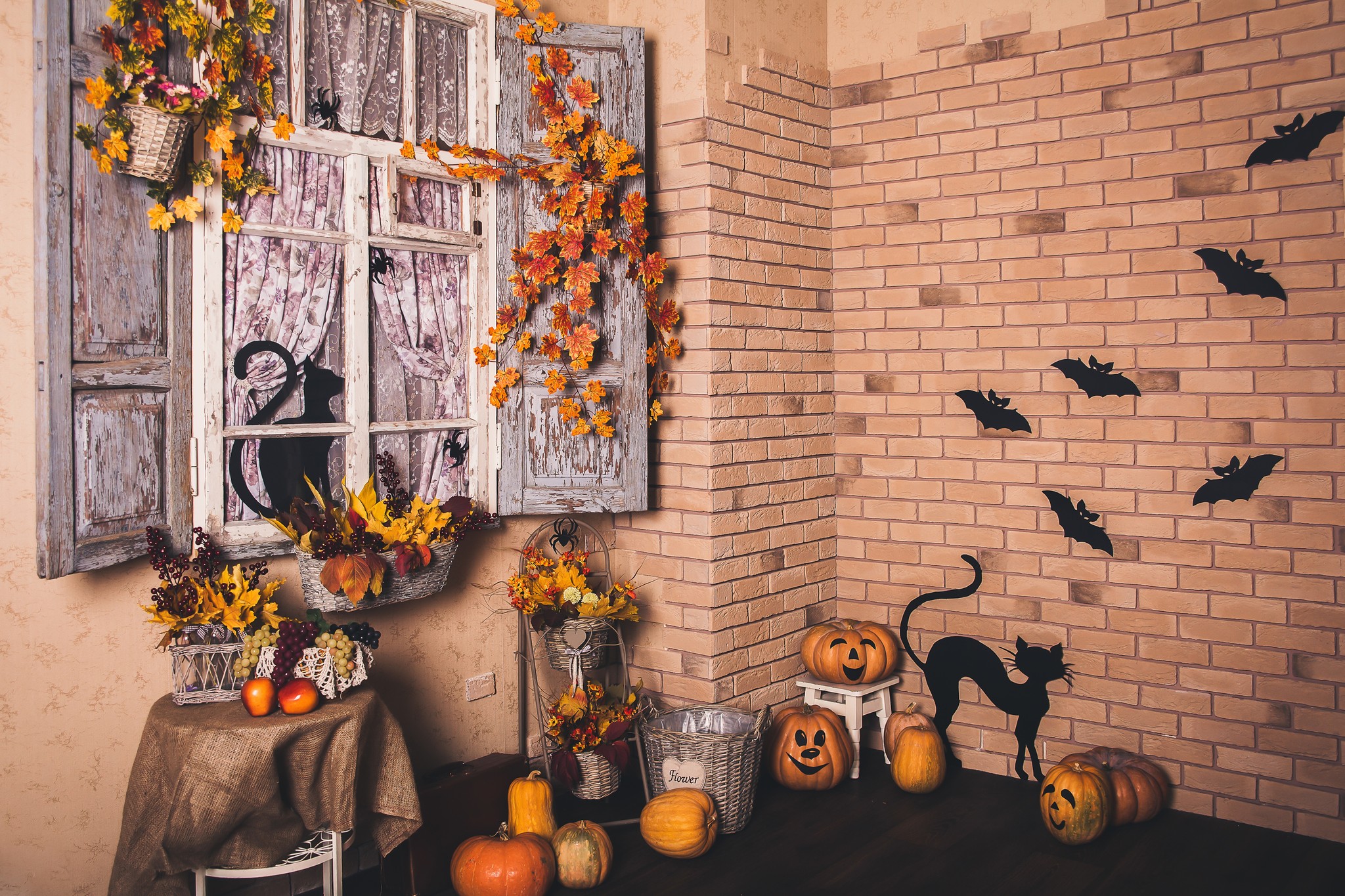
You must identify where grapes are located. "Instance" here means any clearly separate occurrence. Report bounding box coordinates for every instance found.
[233,620,381,689]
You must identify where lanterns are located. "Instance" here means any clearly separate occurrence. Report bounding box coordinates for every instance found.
[1040,760,1112,845]
[766,702,854,791]
[799,618,899,685]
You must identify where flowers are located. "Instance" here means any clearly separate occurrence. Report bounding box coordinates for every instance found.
[468,519,659,637]
[127,62,205,113]
[535,681,658,794]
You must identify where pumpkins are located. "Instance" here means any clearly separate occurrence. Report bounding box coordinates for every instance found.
[450,822,556,896]
[800,618,899,686]
[640,788,719,859]
[1058,747,1167,827]
[763,703,855,791]
[1040,759,1116,846]
[883,702,938,762]
[890,722,946,794]
[550,819,613,889]
[508,770,558,842]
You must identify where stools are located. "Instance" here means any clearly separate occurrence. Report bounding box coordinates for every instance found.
[794,674,900,779]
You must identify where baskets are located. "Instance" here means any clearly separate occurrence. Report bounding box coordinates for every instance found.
[540,614,615,672]
[254,642,376,706]
[636,692,772,836]
[168,642,258,707]
[550,741,624,799]
[116,102,196,187]
[292,539,459,613]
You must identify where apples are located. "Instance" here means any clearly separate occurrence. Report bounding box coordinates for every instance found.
[278,677,319,716]
[241,672,277,717]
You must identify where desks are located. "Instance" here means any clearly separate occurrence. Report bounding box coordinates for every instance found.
[106,683,423,896]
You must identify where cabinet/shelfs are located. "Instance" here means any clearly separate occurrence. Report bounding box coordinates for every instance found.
[515,517,660,854]
